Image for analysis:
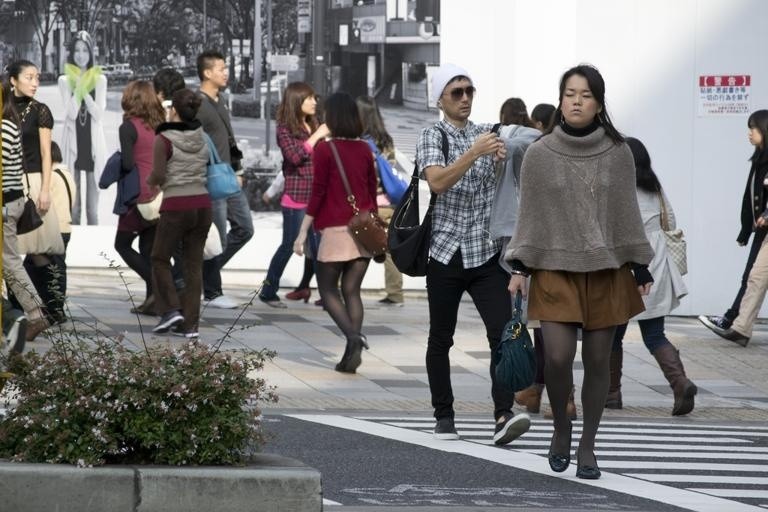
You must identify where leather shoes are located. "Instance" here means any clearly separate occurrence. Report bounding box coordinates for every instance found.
[549,422,572,473]
[259,298,287,310]
[315,299,327,311]
[25,317,51,342]
[575,450,601,481]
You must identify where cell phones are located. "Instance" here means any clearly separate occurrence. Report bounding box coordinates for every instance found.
[490,122,502,134]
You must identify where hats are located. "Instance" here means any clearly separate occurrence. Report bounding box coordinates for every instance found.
[429,63,474,106]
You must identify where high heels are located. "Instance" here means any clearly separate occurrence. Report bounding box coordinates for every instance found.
[286,286,311,304]
[335,334,370,371]
[712,328,751,347]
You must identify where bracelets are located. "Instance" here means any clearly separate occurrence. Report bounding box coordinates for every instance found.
[511,270,528,277]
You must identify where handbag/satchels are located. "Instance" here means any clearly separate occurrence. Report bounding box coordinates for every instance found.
[367,139,410,205]
[490,292,539,393]
[386,124,449,278]
[13,123,44,237]
[323,137,389,264]
[196,91,244,172]
[132,185,166,232]
[196,130,241,202]
[649,181,687,278]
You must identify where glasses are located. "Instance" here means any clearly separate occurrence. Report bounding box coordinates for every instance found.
[442,86,477,102]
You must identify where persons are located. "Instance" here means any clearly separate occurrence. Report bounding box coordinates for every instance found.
[51,140,73,250]
[154,69,185,121]
[354,95,404,307]
[58,30,107,225]
[258,81,329,309]
[1,60,64,324]
[115,79,165,315]
[147,90,210,339]
[531,104,554,135]
[501,98,529,125]
[195,51,254,309]
[415,62,543,446]
[700,107,768,331]
[504,65,654,479]
[286,222,325,307]
[294,92,378,373]
[716,172,768,346]
[604,136,698,417]
[0,294,28,359]
[713,213,764,347]
[0,75,50,341]
[262,95,323,203]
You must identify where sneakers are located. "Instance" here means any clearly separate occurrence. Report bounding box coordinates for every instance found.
[492,415,531,448]
[170,326,201,338]
[150,311,185,333]
[697,314,732,334]
[200,296,239,309]
[4,315,29,358]
[375,297,406,308]
[433,417,460,441]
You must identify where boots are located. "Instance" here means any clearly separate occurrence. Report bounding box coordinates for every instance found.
[604,342,626,410]
[512,382,544,415]
[652,340,698,417]
[542,386,578,422]
